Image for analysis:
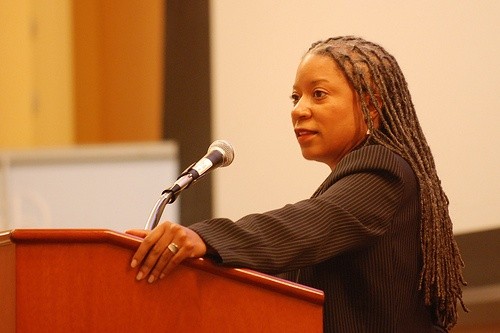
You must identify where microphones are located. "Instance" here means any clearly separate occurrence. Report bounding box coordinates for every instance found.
[162,140,234,204]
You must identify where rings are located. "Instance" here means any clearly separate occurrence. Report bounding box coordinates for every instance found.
[168,243,179,255]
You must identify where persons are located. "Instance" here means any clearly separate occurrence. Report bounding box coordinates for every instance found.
[125,36,472,333]
